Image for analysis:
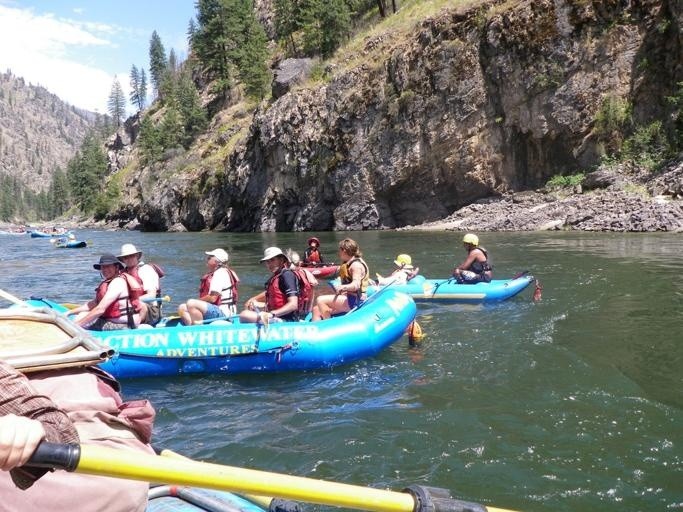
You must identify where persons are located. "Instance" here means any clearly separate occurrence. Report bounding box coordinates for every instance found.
[310,238,370,322]
[452,232,492,285]
[0,360,153,512]
[368,252,413,287]
[303,236,335,268]
[6,221,76,245]
[61,242,319,332]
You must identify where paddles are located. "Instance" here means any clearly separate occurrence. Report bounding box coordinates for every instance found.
[56,295,170,309]
[423,276,456,294]
[251,300,269,330]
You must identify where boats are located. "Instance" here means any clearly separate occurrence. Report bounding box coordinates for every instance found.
[31,231,54,237]
[18,286,415,377]
[364,271,535,303]
[55,240,86,248]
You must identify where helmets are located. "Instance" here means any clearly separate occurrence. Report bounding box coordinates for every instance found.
[463,233,479,245]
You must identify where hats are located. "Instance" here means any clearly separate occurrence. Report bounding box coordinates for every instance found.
[205,248,228,263]
[116,244,142,262]
[393,254,413,269]
[93,254,126,270]
[258,247,288,263]
[308,238,320,247]
